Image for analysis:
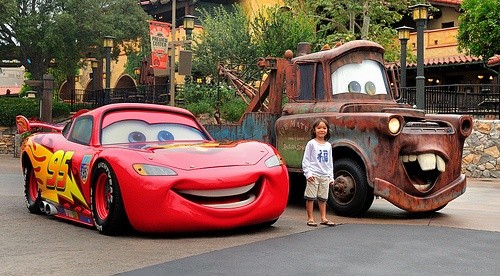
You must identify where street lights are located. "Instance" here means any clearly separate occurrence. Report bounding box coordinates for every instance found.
[181,13,198,102]
[90,59,99,101]
[407,3,431,111]
[395,25,415,98]
[102,34,116,102]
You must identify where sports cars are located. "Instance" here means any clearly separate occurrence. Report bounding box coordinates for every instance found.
[15,102,291,236]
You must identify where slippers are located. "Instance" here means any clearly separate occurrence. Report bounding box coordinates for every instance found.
[320,220,335,226]
[307,221,317,226]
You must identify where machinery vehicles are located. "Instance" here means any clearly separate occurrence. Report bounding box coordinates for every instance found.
[200,38,474,218]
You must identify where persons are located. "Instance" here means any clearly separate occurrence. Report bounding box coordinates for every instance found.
[300,118,336,227]
